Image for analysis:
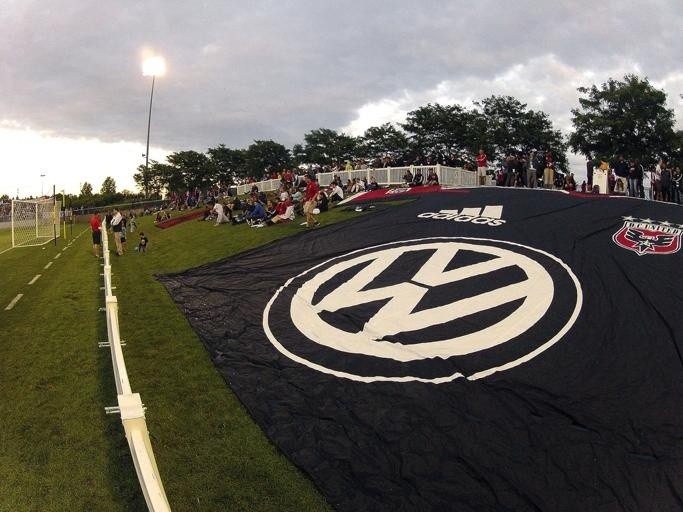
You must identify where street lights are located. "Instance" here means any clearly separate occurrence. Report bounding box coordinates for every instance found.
[142,55,166,199]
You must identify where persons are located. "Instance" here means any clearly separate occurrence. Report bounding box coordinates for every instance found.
[213,164,321,231]
[319,160,353,173]
[402,168,439,187]
[554,151,682,203]
[145,188,233,223]
[90,208,148,260]
[316,174,379,213]
[476,149,555,189]
[355,148,478,172]
